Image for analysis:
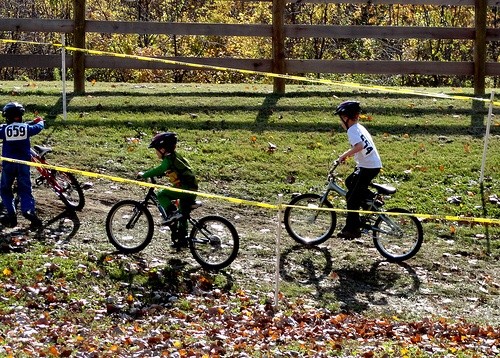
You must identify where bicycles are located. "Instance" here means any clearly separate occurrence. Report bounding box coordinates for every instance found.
[284,161,423,263]
[26,120,86,211]
[105,175,239,270]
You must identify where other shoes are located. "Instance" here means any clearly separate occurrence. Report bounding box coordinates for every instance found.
[5,212,17,220]
[337,231,361,238]
[371,201,385,210]
[20,211,35,218]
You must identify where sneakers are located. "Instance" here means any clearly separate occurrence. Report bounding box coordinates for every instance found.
[171,242,190,251]
[161,211,182,226]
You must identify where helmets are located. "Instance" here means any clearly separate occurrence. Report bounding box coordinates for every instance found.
[334,102,361,115]
[2,101,25,118]
[149,132,177,149]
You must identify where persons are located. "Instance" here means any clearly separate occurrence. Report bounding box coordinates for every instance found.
[136,132,198,248]
[0,102,44,224]
[333,101,382,240]
